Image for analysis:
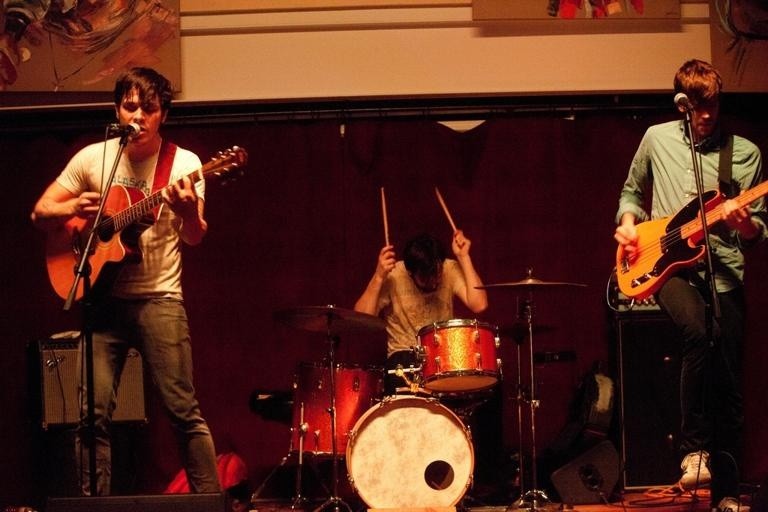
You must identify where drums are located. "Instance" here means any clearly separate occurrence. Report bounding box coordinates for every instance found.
[290,361,386,460]
[345,394,476,512]
[415,319,504,395]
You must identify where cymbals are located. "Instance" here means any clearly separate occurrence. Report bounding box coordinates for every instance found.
[473,279,588,291]
[497,322,550,338]
[271,305,385,334]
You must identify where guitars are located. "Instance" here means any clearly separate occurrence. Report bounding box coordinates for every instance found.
[616,179,767,301]
[46,145,249,302]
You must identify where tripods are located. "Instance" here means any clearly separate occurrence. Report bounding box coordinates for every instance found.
[313,334,352,512]
[249,400,329,510]
[507,344,548,508]
[503,302,563,512]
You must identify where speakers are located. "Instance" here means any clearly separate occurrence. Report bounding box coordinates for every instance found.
[549,424,627,505]
[607,311,682,490]
[45,493,231,512]
[26,330,150,431]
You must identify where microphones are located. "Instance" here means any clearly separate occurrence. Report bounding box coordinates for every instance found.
[110,123,141,137]
[674,93,696,112]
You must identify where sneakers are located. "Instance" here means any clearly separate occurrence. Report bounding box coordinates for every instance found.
[680,450,711,491]
[713,494,750,512]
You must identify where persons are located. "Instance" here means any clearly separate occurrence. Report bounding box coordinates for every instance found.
[31,68,222,497]
[614,60,768,511]
[352,231,489,398]
